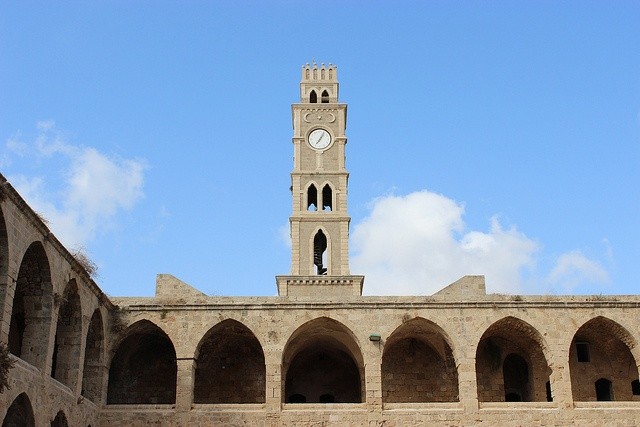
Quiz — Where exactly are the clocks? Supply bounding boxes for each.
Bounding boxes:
[307,127,333,150]
